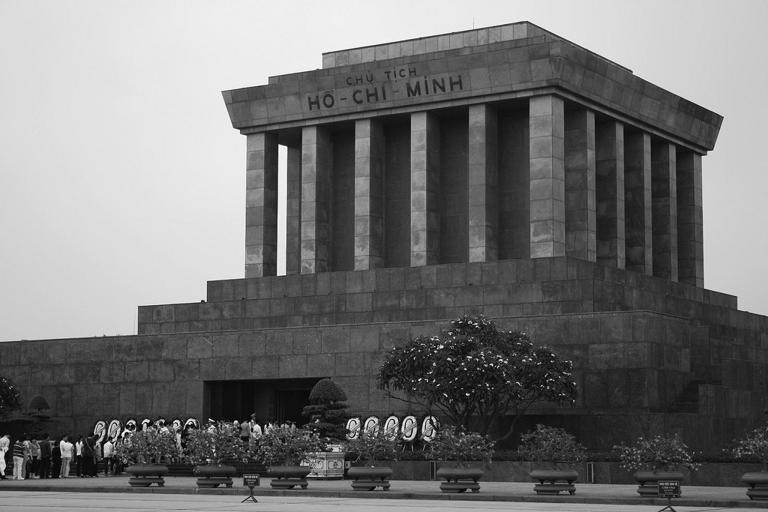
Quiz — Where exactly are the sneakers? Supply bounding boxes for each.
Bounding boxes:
[0,475,40,480]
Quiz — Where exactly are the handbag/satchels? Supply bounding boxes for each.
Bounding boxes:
[91,450,96,455]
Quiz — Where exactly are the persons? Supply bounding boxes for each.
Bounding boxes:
[1,408,299,489]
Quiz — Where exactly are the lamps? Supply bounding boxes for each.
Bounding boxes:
[656,480,682,512]
[238,473,260,503]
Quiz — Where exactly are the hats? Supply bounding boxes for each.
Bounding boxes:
[250,413,257,417]
[208,418,215,423]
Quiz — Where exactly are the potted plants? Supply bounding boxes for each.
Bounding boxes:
[120,428,178,488]
[189,425,241,490]
[611,431,703,498]
[299,378,350,480]
[265,442,311,491]
[421,424,497,493]
[513,421,587,496]
[721,424,767,500]
[348,429,399,495]
